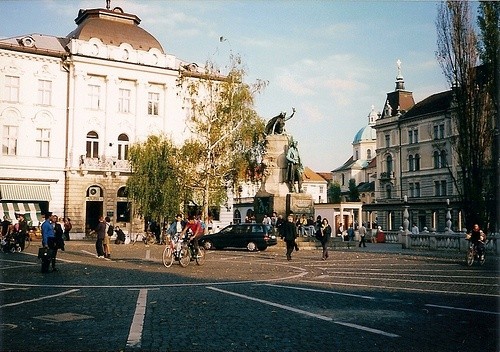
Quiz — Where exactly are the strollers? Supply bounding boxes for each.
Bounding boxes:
[0,230,22,254]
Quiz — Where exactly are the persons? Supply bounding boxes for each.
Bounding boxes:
[467,224,484,261]
[270,107,304,193]
[0,211,419,272]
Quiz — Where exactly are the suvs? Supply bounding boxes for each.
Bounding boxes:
[197,223,278,252]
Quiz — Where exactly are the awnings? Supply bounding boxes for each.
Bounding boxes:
[0,184,52,202]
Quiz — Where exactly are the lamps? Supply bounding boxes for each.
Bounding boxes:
[81,155,85,164]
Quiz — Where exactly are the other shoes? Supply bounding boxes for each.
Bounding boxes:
[325,256,329,259]
[322,258,325,260]
[286,254,291,260]
[104,258,111,261]
[96,255,104,258]
[481,255,484,260]
[53,268,59,271]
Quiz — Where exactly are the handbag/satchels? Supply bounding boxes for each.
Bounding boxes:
[38,248,51,259]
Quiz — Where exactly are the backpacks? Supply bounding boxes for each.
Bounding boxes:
[106,223,113,236]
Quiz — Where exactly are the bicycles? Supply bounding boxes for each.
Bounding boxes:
[162,232,207,268]
[465,238,486,266]
